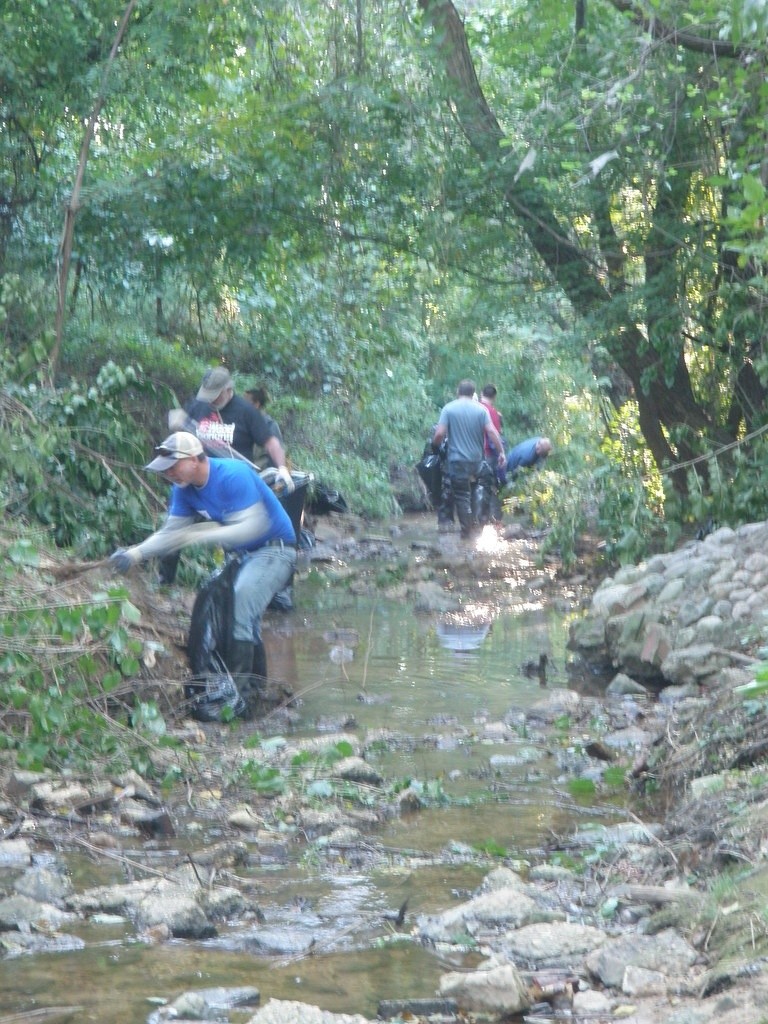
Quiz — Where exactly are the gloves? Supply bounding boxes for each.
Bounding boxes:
[109,546,140,574]
[275,465,295,494]
[168,409,188,432]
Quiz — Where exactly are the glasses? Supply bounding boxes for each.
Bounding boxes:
[154,446,192,457]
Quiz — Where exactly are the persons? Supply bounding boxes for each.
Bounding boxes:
[107,367,296,722]
[435,374,554,540]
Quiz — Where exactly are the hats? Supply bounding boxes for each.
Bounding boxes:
[142,431,203,471]
[195,366,230,403]
